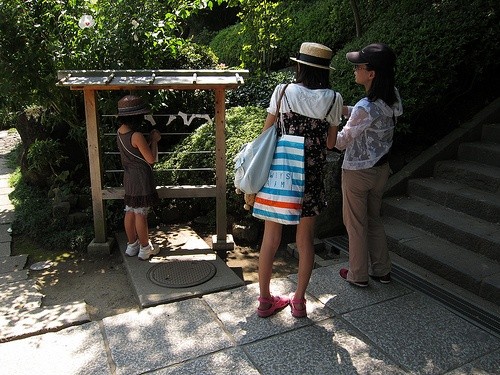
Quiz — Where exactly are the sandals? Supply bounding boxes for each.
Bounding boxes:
[289,292,307,317]
[258,295,290,318]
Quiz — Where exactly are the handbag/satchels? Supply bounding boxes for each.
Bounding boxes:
[233,124,277,194]
[252,134,305,225]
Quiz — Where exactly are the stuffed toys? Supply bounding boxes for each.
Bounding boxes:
[235,189,256,210]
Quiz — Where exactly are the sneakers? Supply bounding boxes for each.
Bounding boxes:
[125,238,140,257]
[138,241,160,261]
[340,269,368,286]
[369,272,393,283]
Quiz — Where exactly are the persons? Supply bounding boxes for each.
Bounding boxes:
[335,43,402,287]
[116,95,160,259]
[257,42,343,319]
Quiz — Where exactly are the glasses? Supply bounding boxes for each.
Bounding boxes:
[355,65,368,71]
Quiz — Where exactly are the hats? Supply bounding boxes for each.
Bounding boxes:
[290,41,337,71]
[346,43,396,72]
[114,95,155,116]
